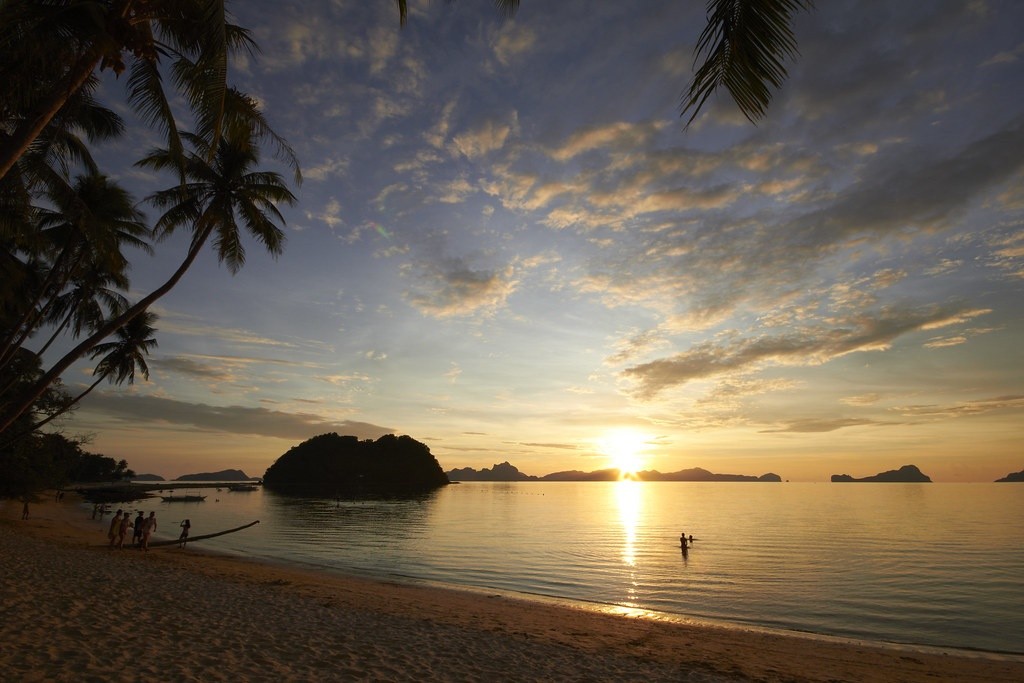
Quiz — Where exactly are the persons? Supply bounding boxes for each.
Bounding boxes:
[689,535,693,542]
[681,532,689,551]
[140,512,158,552]
[178,519,191,551]
[99,501,107,521]
[108,509,123,550]
[93,501,102,520]
[22,497,33,520]
[116,512,134,551]
[54,490,66,505]
[132,511,146,548]
